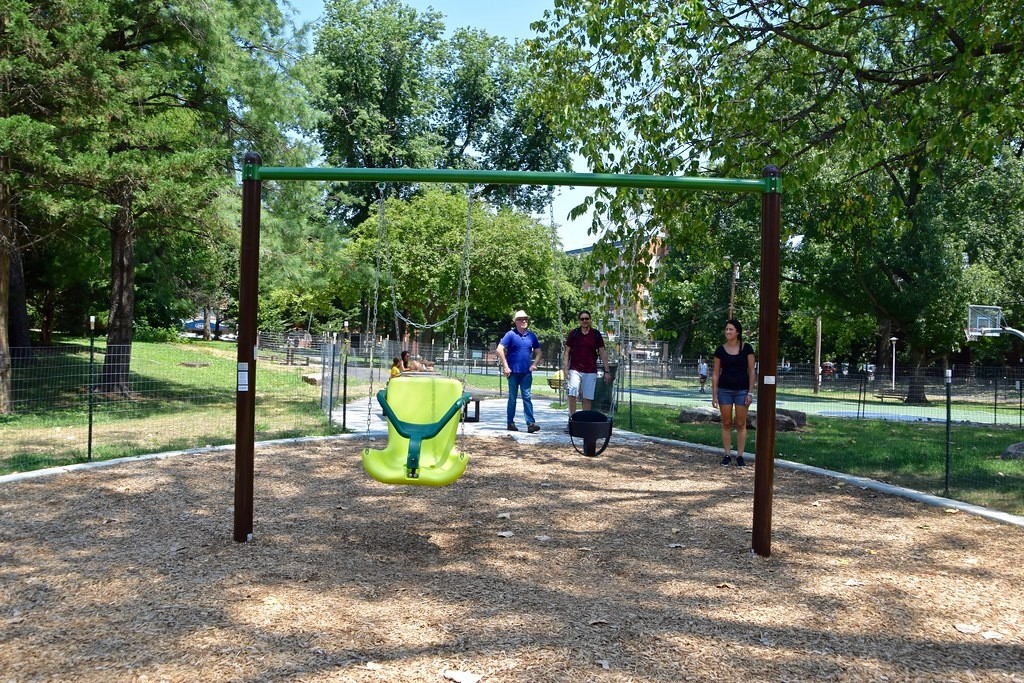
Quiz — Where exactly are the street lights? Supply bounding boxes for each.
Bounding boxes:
[889,336,899,392]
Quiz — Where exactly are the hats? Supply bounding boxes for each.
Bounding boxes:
[513,310,530,322]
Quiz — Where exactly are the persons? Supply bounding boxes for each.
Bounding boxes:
[698,358,709,394]
[868,366,874,373]
[390,352,435,378]
[288,327,312,348]
[496,310,542,433]
[713,319,755,467]
[564,311,611,433]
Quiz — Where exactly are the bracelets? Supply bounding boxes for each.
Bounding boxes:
[605,371,610,374]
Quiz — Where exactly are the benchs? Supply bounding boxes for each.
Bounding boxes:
[546,378,565,393]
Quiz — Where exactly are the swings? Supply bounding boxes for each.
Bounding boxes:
[360,189,474,486]
[545,190,646,457]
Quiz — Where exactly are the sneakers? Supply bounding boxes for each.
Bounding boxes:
[736,456,747,468]
[720,455,731,466]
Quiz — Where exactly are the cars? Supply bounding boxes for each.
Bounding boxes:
[822,360,875,379]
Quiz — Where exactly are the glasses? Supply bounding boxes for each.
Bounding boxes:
[517,319,528,323]
[579,317,590,321]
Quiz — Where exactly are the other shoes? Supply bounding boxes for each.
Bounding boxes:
[528,423,540,433]
[507,423,518,431]
[700,390,705,394]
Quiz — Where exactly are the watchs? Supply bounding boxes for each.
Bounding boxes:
[748,393,754,397]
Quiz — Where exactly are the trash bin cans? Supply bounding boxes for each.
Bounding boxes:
[582,361,618,412]
